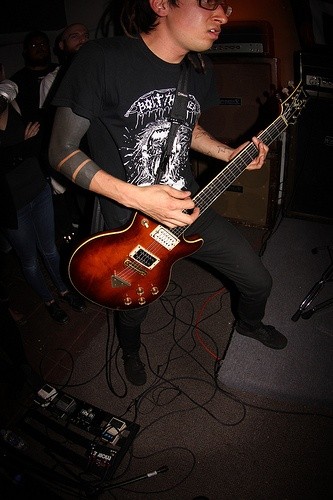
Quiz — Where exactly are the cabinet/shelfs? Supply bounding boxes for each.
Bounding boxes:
[190,58,279,228]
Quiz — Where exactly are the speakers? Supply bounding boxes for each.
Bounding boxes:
[189,148,276,231]
[274,90,333,225]
[198,57,277,149]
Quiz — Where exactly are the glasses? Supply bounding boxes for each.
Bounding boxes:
[198,0,232,17]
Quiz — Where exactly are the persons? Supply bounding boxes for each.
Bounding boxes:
[0,62,87,325]
[36,10,118,311]
[21,32,60,83]
[45,0,290,385]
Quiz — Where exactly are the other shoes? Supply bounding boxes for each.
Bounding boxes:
[122,348,146,386]
[45,299,67,322]
[235,320,288,350]
[60,289,86,313]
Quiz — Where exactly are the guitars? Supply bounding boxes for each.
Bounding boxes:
[67,76,308,312]
